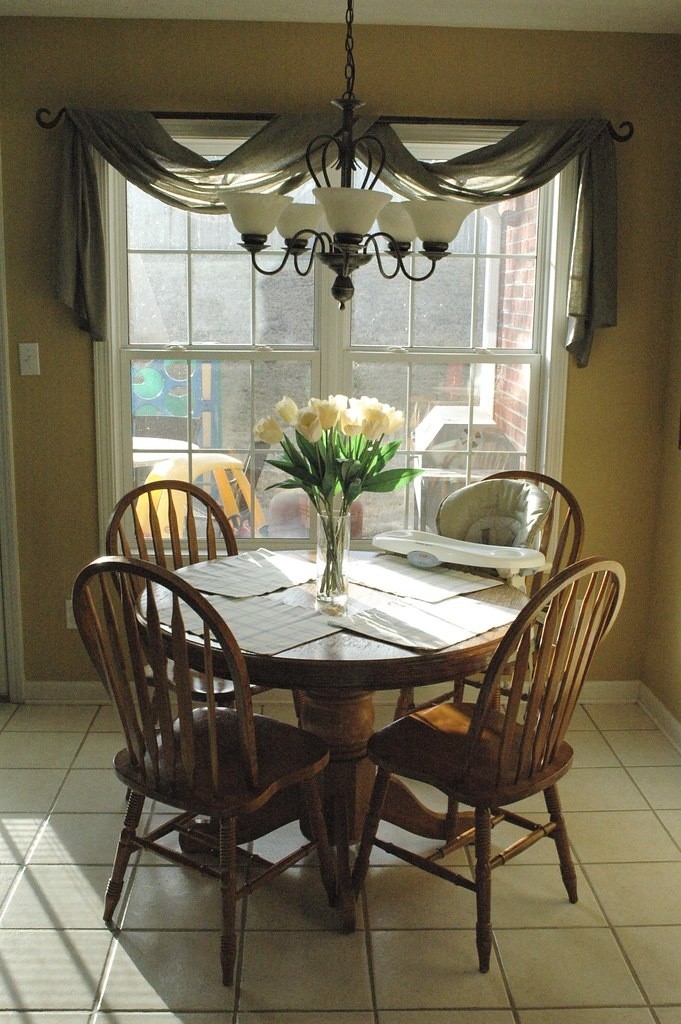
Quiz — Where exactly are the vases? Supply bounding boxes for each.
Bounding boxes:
[315,509,351,614]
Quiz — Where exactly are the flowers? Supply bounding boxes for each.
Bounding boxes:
[251,390,425,597]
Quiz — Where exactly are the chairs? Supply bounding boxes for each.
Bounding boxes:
[69,554,342,987]
[104,478,272,716]
[391,470,585,724]
[349,555,627,974]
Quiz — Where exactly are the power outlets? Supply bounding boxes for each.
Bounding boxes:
[18,342,40,376]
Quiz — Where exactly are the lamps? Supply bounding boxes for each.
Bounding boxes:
[218,0,477,313]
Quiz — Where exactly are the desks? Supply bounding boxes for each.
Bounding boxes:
[135,547,538,848]
